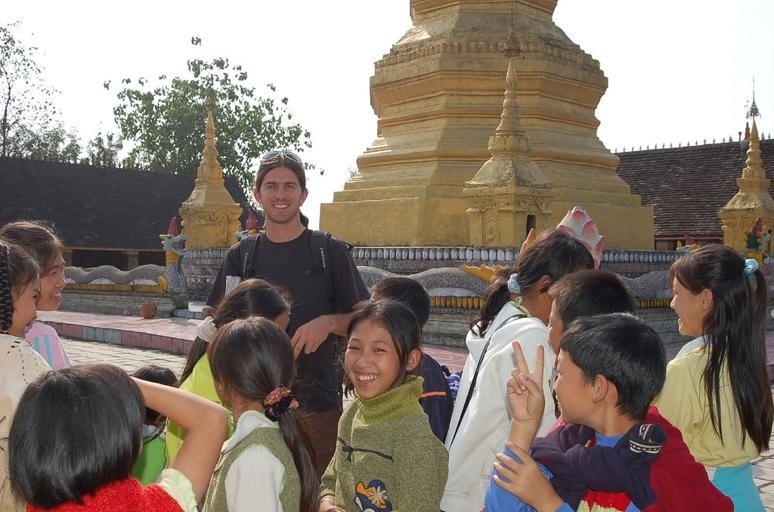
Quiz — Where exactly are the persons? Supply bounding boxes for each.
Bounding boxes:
[205,148,373,474]
[0,219,774,512]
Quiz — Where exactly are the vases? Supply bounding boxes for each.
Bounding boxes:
[140,304,157,319]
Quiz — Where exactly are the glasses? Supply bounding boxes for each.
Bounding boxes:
[260,151,302,167]
